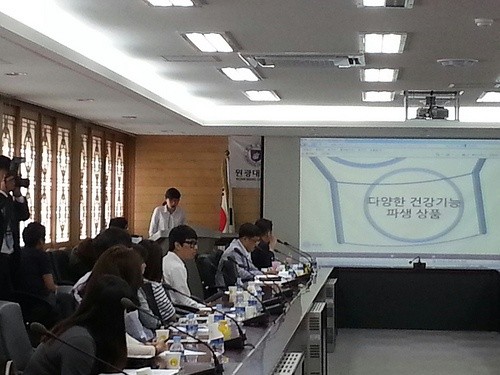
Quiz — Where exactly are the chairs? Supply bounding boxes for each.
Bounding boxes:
[0,245,224,375]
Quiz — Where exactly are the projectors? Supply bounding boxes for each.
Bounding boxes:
[416,108,449,119]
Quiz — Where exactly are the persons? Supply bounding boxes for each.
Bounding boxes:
[214,222,263,292]
[24,275,134,375]
[5,221,55,345]
[148,187,185,238]
[72,216,177,361]
[252,218,276,269]
[161,223,212,315]
[0,154,29,265]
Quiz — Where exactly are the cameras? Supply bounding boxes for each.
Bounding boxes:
[4,157,30,188]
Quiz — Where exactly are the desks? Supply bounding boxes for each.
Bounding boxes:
[329,267,500,331]
[148,267,338,375]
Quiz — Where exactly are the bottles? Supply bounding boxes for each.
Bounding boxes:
[186,313,199,339]
[235,292,245,317]
[214,303,225,322]
[288,269,295,280]
[169,336,185,369]
[218,319,229,341]
[256,283,263,310]
[246,282,258,318]
[235,278,244,291]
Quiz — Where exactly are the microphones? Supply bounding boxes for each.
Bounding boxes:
[120,239,313,375]
[29,322,128,375]
[409,256,426,269]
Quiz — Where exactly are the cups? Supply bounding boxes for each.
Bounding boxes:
[155,286,255,370]
[272,262,280,271]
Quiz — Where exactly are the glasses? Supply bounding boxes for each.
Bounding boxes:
[247,237,261,245]
[166,197,180,202]
[184,241,199,248]
[125,225,129,231]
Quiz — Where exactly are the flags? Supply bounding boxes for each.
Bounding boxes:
[227,135,261,187]
[218,150,236,234]
